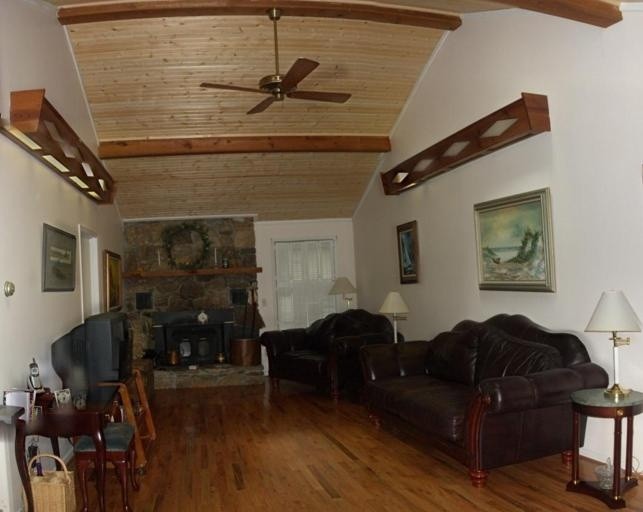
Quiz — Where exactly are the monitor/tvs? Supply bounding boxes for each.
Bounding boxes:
[52,312,132,389]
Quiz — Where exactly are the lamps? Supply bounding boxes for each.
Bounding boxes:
[378,291,412,345]
[583,288,641,400]
[327,277,360,308]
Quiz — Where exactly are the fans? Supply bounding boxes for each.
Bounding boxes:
[198,4,354,116]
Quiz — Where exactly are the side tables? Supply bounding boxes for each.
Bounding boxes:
[565,387,643,511]
[10,384,123,512]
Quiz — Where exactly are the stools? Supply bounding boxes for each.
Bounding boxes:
[72,420,141,512]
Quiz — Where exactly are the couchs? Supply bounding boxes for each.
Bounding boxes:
[358,312,610,488]
[257,307,406,401]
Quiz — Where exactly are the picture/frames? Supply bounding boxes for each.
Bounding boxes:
[473,187,556,293]
[397,220,419,284]
[41,222,76,292]
[103,249,122,312]
